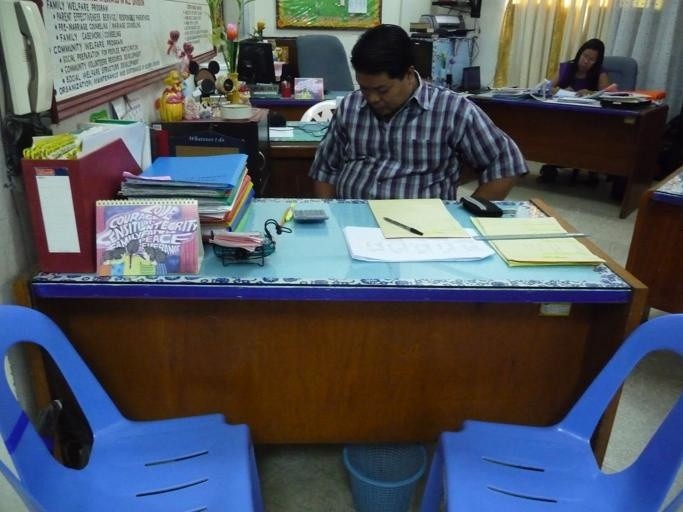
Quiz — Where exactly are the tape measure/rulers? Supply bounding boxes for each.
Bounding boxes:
[473,232,590,241]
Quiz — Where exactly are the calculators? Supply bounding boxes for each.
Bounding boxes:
[293,209,328,223]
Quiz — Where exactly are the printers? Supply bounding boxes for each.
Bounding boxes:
[420,14,476,38]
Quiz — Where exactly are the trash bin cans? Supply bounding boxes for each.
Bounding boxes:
[342,443,428,512]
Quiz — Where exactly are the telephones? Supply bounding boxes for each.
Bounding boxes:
[0,0,54,116]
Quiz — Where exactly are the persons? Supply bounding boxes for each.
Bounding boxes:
[539,39,610,177]
[308,24,529,200]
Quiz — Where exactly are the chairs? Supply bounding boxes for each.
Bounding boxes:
[0,305,266,512]
[296,34,356,91]
[420,314,683,512]
[601,56,638,91]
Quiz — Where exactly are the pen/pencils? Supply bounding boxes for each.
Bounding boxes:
[383,217,423,235]
[284,202,295,222]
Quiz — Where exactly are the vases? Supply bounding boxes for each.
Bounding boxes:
[227,72,241,104]
[440,67,447,80]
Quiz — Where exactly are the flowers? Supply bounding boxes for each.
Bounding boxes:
[438,52,455,68]
[203,11,240,73]
[247,20,266,42]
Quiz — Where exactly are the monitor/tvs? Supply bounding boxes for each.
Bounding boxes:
[230,40,277,86]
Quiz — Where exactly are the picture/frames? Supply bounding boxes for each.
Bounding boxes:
[276,0,382,30]
